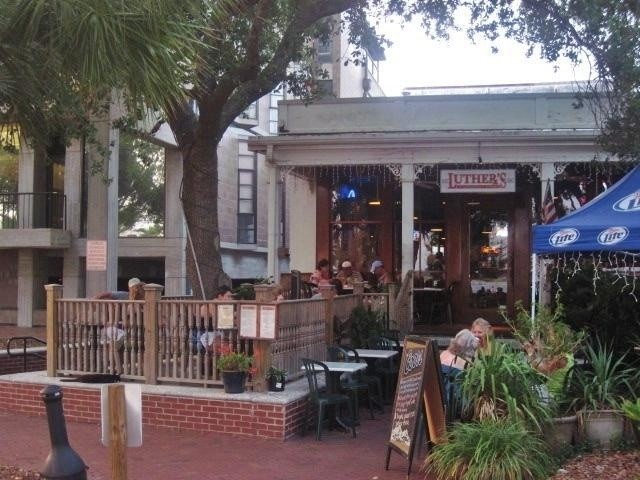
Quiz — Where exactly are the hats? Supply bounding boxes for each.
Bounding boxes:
[369,260,384,272]
[342,260,352,268]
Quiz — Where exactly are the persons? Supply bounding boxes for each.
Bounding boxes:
[438,326,480,420]
[184,284,235,376]
[92,324,129,376]
[470,317,493,351]
[519,322,576,411]
[273,255,396,353]
[92,276,147,353]
[413,245,507,310]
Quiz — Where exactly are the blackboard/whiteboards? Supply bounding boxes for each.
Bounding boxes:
[388,335,434,458]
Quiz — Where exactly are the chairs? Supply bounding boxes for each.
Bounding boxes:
[303,282,318,298]
[429,280,460,328]
[300,329,405,441]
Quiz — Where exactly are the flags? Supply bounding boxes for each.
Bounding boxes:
[541,178,560,225]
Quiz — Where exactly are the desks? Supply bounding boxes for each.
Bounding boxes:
[413,287,445,324]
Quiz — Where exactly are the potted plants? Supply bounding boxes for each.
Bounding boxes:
[264,365,289,391]
[253,273,279,301]
[540,328,640,452]
[217,351,254,393]
